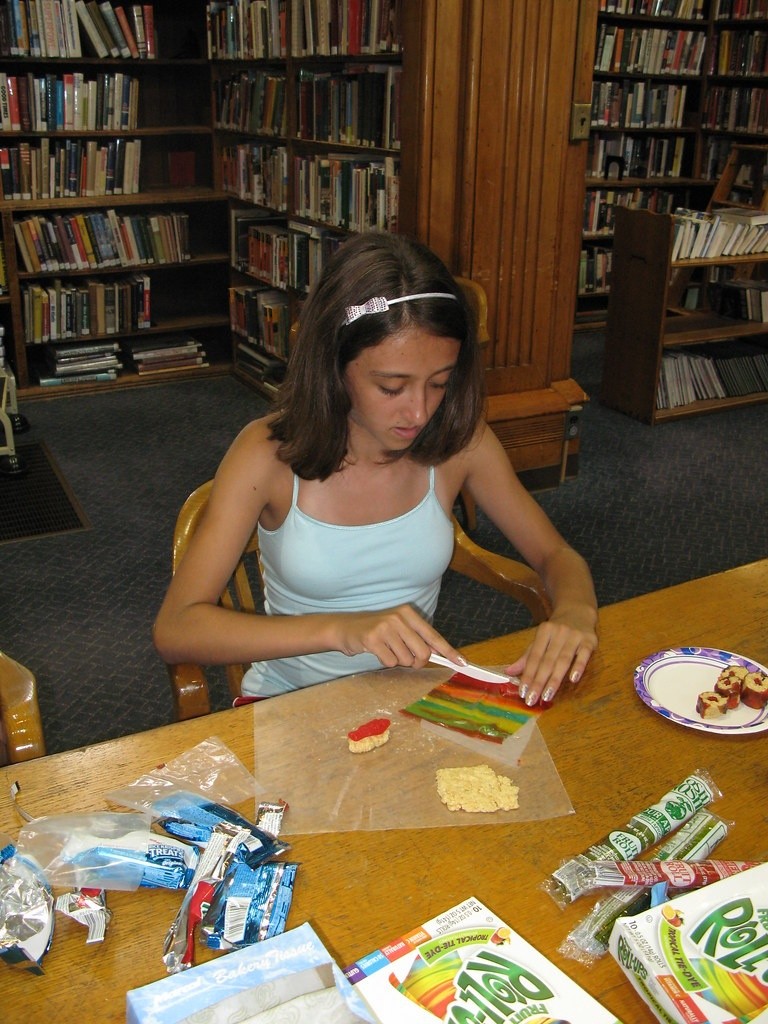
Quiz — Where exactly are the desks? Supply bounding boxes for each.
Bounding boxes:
[0,561,768,1024]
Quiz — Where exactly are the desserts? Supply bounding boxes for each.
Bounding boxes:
[348,719,390,753]
[697,665,768,720]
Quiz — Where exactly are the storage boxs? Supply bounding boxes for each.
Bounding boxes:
[341,896,623,1024]
[126,921,377,1024]
[609,861,768,1024]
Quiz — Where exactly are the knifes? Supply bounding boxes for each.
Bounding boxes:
[428,652,510,684]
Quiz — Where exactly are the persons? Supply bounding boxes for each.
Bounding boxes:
[154,234,597,707]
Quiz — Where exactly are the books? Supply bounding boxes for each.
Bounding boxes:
[0,0,208,387]
[577,1,768,409]
[206,0,401,393]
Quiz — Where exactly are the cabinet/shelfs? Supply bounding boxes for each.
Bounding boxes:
[571,0,712,335]
[0,0,233,406]
[206,0,436,404]
[699,19,768,193]
[596,203,768,427]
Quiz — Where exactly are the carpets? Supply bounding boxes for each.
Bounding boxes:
[0,439,98,546]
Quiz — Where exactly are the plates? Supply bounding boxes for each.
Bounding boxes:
[633,647,768,735]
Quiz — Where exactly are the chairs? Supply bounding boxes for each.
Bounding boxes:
[167,479,553,722]
